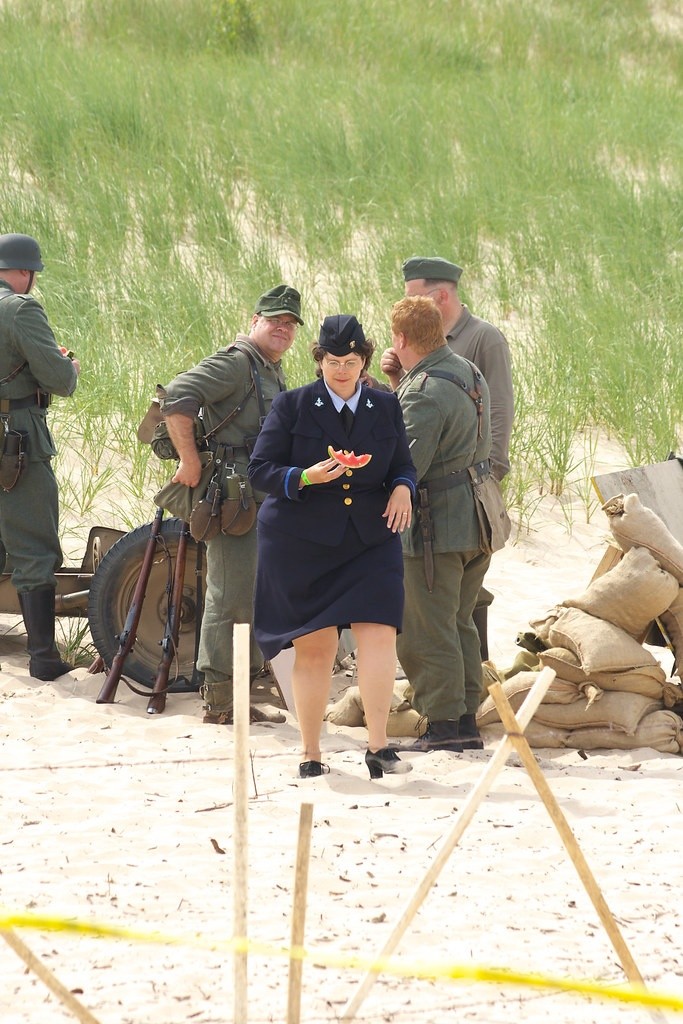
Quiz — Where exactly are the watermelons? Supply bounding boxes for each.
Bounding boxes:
[328,445,372,469]
[58,346,75,358]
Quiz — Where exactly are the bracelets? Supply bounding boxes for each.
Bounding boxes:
[301,470,313,486]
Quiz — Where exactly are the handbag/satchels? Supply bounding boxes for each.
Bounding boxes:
[150,415,206,459]
[153,451,215,522]
[468,467,511,555]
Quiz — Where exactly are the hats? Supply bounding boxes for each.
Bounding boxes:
[255,284,304,326]
[319,315,365,357]
[402,256,462,281]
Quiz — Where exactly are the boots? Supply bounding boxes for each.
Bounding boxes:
[18,586,76,681]
[200,679,286,725]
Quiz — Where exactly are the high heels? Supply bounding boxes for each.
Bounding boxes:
[364,746,412,779]
[299,760,331,778]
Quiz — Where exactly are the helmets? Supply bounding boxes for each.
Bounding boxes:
[0,233,43,271]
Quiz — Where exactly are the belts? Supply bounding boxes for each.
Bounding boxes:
[9,392,52,410]
[415,458,489,494]
[209,444,248,458]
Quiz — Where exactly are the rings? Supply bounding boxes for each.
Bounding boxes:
[403,512,408,515]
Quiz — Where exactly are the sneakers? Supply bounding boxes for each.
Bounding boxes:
[389,720,463,752]
[459,714,484,749]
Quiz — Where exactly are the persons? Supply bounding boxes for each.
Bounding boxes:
[361,257,513,752]
[161,286,304,726]
[0,234,80,682]
[248,315,416,779]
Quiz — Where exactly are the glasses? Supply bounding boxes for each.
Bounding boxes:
[264,318,298,330]
[323,360,361,370]
